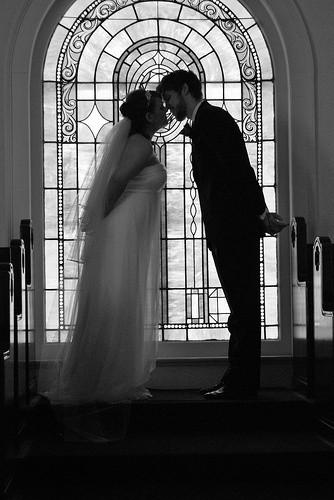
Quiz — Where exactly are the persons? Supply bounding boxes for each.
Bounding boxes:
[36,90,169,406]
[156,69,290,403]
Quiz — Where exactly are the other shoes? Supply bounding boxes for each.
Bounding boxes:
[199,380,256,399]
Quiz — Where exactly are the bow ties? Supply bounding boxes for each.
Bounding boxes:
[179,122,198,138]
